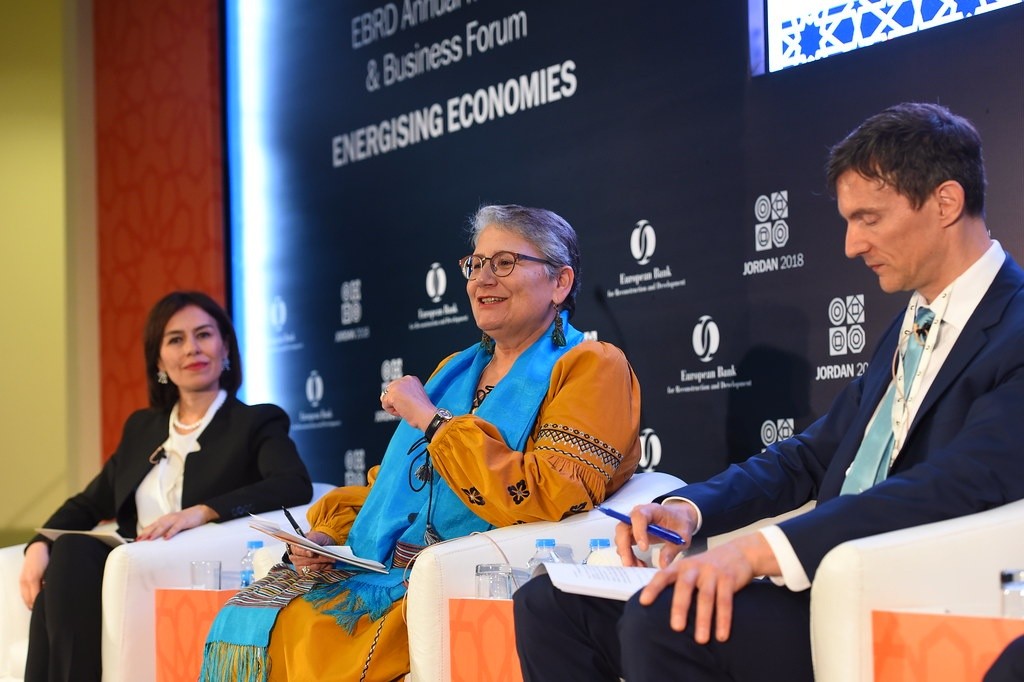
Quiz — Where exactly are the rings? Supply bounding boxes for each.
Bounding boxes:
[301,565,310,574]
[285,543,292,555]
[381,389,388,397]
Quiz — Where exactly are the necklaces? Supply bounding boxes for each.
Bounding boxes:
[174,415,203,430]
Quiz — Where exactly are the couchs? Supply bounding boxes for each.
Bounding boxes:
[0,478,336,677]
[101,470,685,682]
[587,496,1024,682]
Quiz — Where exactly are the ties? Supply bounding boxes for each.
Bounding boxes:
[840,306,936,498]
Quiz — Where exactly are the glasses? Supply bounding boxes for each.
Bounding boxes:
[458,251,557,280]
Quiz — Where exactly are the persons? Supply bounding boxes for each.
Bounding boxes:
[201,203,639,682]
[20,291,313,682]
[512,103,1024,682]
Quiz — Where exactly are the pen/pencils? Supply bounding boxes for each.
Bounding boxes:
[593,503,688,546]
[281,505,307,539]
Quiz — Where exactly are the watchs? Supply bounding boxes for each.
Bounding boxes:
[424,408,453,441]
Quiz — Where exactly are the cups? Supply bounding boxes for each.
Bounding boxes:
[190,561,222,590]
[1002,571,1024,619]
[476,565,512,601]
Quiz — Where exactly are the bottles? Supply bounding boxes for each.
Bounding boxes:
[241,541,264,589]
[527,538,565,576]
[583,539,612,568]
